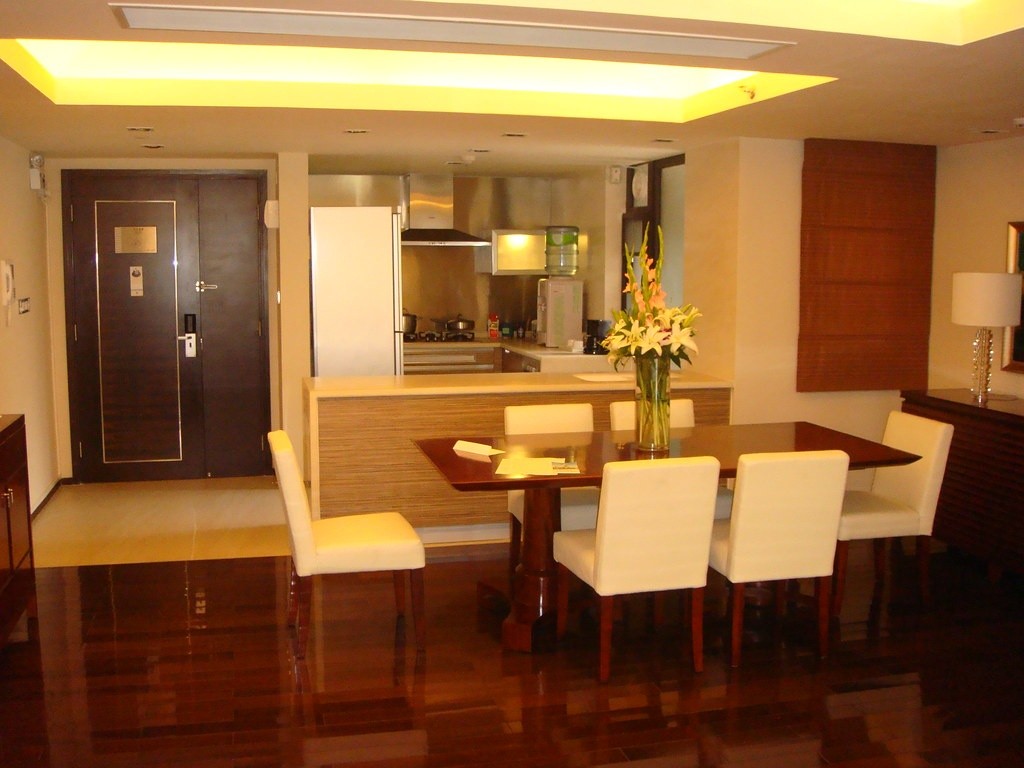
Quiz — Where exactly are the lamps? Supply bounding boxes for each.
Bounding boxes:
[952,272,1023,408]
[29,151,50,205]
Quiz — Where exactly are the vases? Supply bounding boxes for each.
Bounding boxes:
[636,355,672,453]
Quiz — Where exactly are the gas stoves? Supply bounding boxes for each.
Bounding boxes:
[403,332,478,346]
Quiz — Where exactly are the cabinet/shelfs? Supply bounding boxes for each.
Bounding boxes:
[403,348,542,376]
[901,388,1024,561]
[0,412,35,592]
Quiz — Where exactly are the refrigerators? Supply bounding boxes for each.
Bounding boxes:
[310,204,404,377]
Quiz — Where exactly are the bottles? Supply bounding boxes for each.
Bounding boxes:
[487,312,499,340]
[545,225,579,276]
[516,323,526,338]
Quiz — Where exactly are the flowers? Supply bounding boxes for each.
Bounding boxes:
[602,219,703,371]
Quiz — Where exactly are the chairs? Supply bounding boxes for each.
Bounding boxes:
[608,400,735,521]
[830,412,955,616]
[268,430,426,660]
[686,450,852,666]
[503,403,602,582]
[552,455,720,681]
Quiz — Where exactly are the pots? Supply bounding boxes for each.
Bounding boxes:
[401,307,423,334]
[430,313,475,332]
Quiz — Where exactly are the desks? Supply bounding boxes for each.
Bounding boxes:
[410,422,922,655]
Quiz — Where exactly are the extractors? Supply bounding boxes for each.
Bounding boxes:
[401,173,492,247]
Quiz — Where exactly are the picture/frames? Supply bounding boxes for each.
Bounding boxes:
[1002,220,1024,373]
